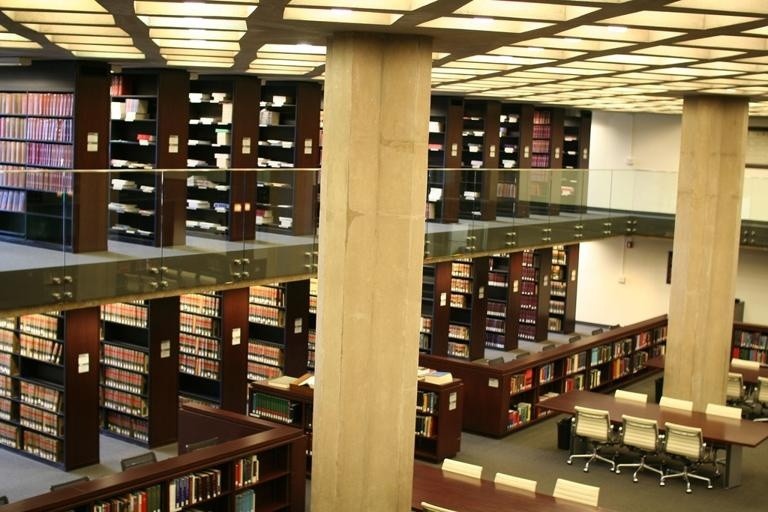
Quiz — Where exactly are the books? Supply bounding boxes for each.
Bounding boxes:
[179,292,221,380]
[186,92,233,232]
[1,93,74,212]
[461,116,485,216]
[560,135,580,196]
[249,282,284,327]
[507,352,584,430]
[416,367,453,436]
[0,307,63,463]
[578,321,768,392]
[486,246,566,349]
[309,278,318,313]
[256,96,294,229]
[419,258,472,358]
[247,340,315,424]
[530,110,552,196]
[425,121,444,220]
[99,300,150,442]
[308,330,317,368]
[109,99,156,237]
[497,114,519,197]
[88,451,265,511]
